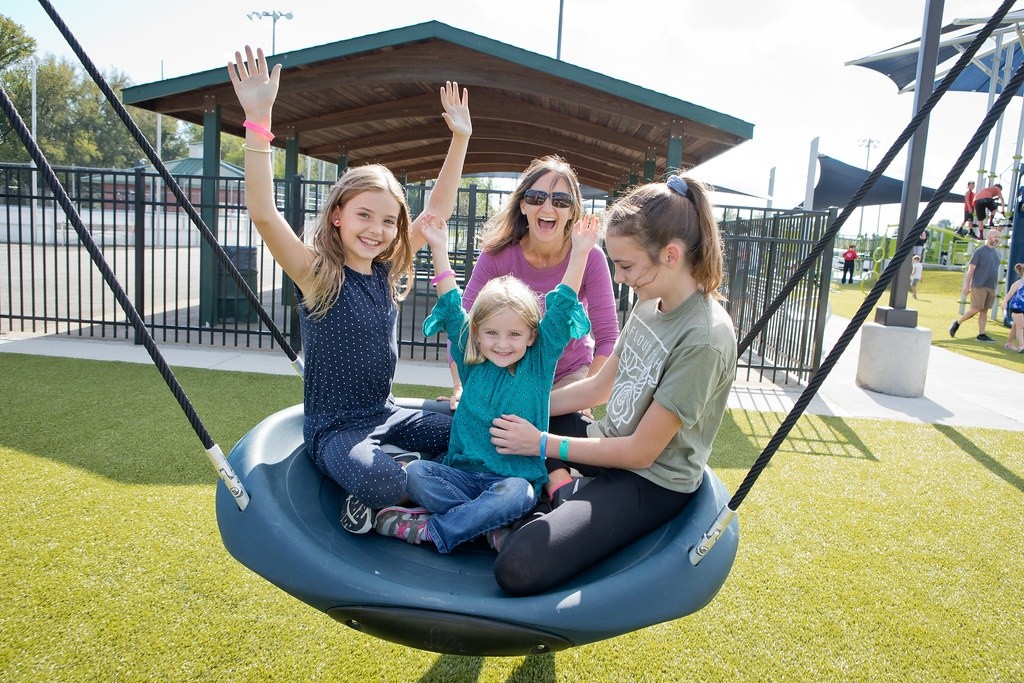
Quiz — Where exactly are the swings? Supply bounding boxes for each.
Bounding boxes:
[0,0,1024,659]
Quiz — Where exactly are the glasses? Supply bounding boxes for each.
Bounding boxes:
[522,189,572,208]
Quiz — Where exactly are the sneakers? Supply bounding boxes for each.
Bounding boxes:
[485,528,512,554]
[372,506,438,544]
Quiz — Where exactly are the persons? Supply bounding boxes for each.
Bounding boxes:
[972,184,1004,240]
[436,153,620,420]
[479,174,739,596]
[228,45,472,534]
[912,232,926,261]
[841,244,858,283]
[949,229,1001,342]
[910,255,922,299]
[373,213,601,552]
[955,181,976,235]
[1001,263,1024,353]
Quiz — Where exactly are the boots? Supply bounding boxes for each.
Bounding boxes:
[977,230,984,239]
[989,215,994,226]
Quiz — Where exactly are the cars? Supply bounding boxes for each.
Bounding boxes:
[831,247,847,271]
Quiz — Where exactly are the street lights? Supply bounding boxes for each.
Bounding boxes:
[856,138,881,238]
[246,11,296,178]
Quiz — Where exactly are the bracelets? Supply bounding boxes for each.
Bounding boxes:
[242,120,275,141]
[559,438,569,461]
[539,431,547,460]
[432,270,457,287]
[241,144,272,153]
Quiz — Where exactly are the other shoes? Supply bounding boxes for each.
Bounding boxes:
[966,232,977,238]
[955,230,964,237]
[1005,344,1024,353]
[537,475,595,511]
[975,333,995,344]
[950,320,960,337]
[339,492,377,535]
